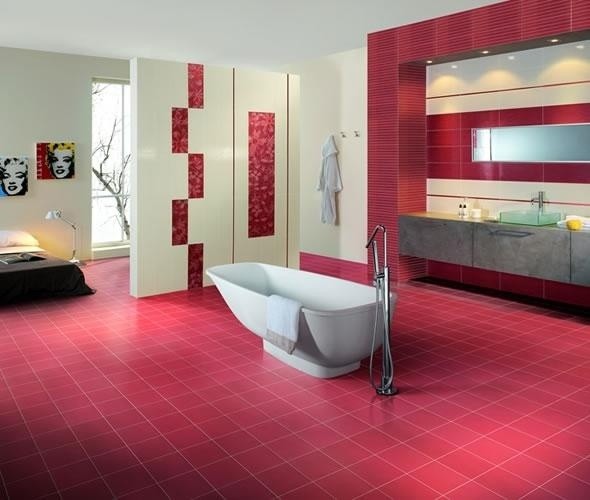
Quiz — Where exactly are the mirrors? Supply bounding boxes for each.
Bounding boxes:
[469,124,590,164]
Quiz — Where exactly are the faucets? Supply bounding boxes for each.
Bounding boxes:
[531,192,543,208]
[366,224,388,266]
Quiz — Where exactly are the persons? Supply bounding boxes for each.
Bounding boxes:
[46,143,74,179]
[0,156,27,196]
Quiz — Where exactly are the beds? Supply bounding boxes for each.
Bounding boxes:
[0,230,87,306]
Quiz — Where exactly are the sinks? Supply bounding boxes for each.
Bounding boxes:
[500,211,560,226]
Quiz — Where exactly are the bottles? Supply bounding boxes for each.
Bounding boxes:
[458,201,468,219]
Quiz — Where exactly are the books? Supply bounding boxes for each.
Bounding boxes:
[0,252,47,265]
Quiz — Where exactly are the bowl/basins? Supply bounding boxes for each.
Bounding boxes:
[566,220,581,231]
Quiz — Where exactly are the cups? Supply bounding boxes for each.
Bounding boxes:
[471,209,481,219]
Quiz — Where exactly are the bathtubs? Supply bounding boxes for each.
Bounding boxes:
[205,262,397,379]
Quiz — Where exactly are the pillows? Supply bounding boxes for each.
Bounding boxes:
[260,293,301,354]
[0,230,39,248]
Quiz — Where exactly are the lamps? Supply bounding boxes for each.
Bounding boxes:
[44,210,81,263]
